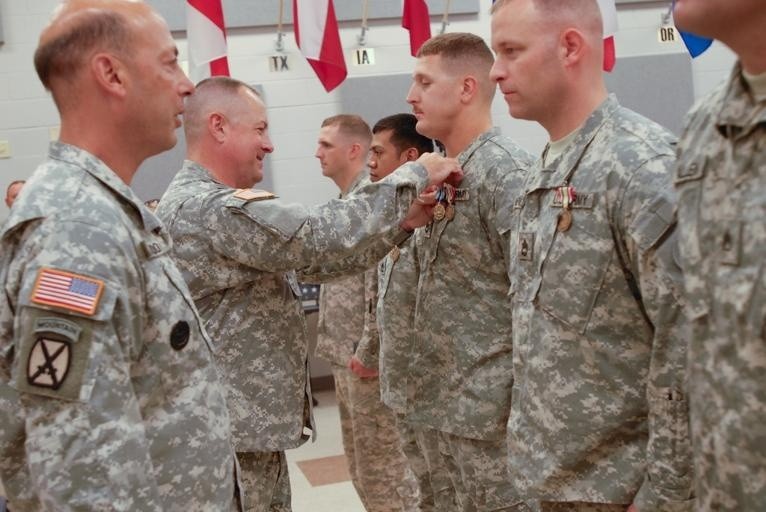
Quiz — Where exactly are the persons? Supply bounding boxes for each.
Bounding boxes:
[368,111,459,511]
[4,176,28,208]
[487,0,698,511]
[0,0,246,512]
[152,75,465,511]
[311,113,423,510]
[670,1,766,511]
[405,30,539,512]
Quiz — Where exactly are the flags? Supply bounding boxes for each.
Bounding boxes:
[596,0,619,73]
[292,0,349,94]
[401,0,432,58]
[672,0,716,60]
[183,1,231,90]
